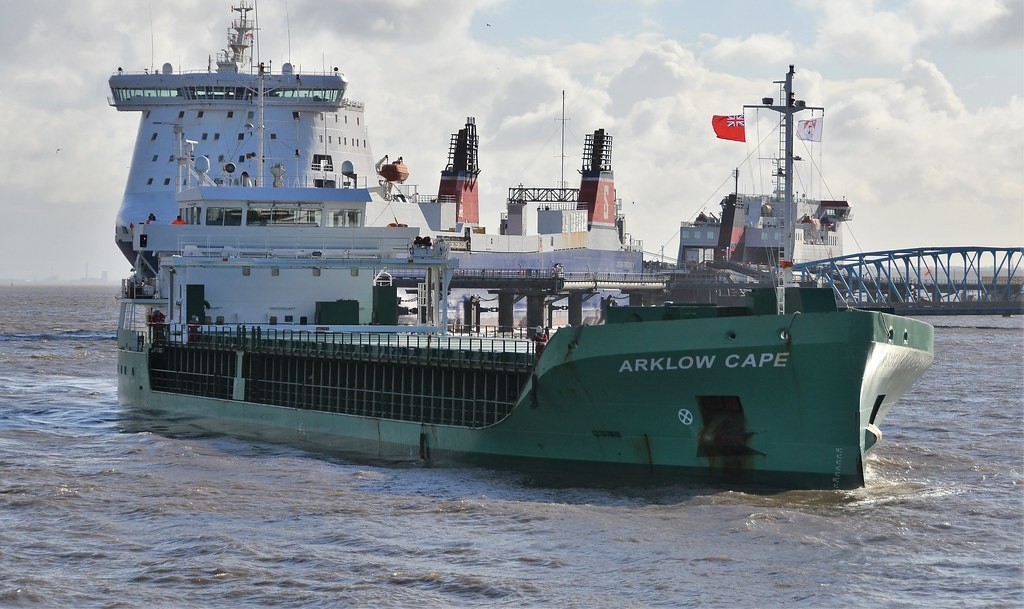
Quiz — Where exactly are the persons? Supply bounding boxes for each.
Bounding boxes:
[188,315,200,342]
[146,213,156,224]
[150,310,166,339]
[172,215,185,224]
[530,326,548,357]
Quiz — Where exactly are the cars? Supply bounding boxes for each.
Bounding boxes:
[106,2,937,489]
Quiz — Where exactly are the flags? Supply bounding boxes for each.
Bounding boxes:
[795,117,824,142]
[712,115,746,143]
